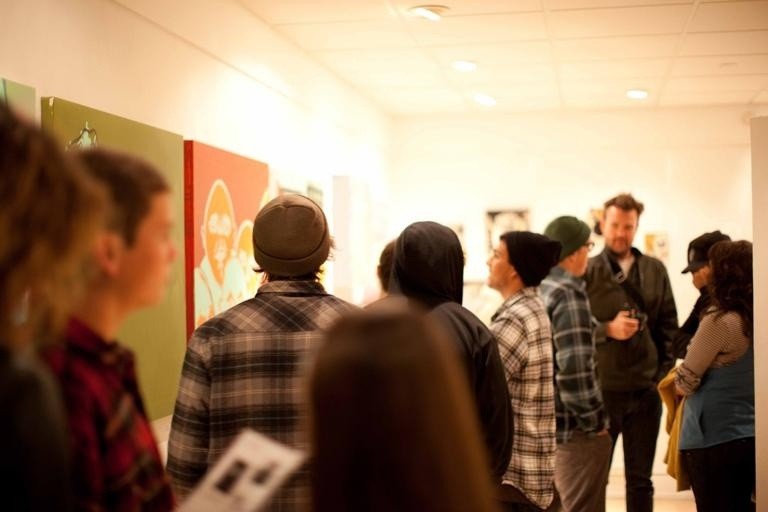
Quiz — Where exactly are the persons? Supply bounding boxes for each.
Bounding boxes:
[672,239,753,512]
[359,242,413,318]
[669,231,731,367]
[308,313,535,509]
[582,195,679,512]
[1,99,113,511]
[487,232,564,508]
[166,193,363,511]
[38,149,179,509]
[363,222,514,479]
[538,216,613,512]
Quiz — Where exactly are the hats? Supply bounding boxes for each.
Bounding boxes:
[250,193,331,277]
[503,229,564,288]
[681,229,733,275]
[542,216,592,263]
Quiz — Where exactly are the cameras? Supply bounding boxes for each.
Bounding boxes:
[625,306,648,331]
